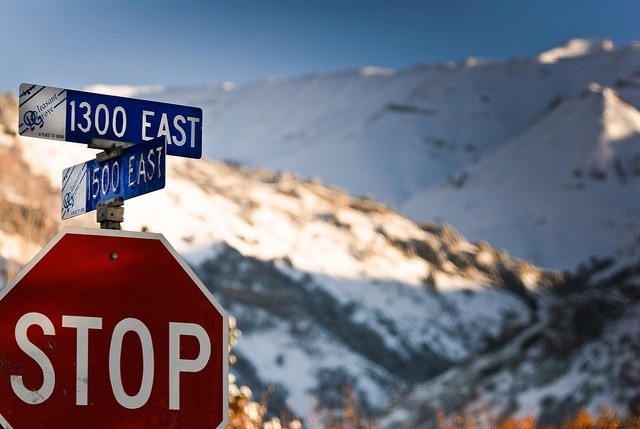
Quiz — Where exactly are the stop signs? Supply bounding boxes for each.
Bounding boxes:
[0,227,228,429]
